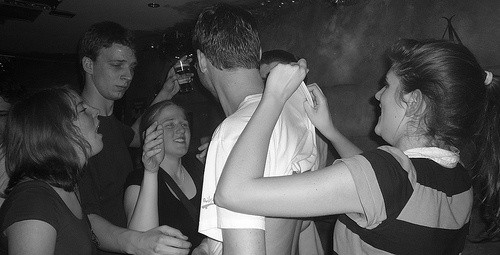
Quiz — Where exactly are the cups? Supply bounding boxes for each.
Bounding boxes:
[174,59,193,92]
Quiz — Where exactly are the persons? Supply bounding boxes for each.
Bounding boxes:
[195,5,328,255]
[123,101,210,255]
[215,40,500,255]
[0,25,193,255]
